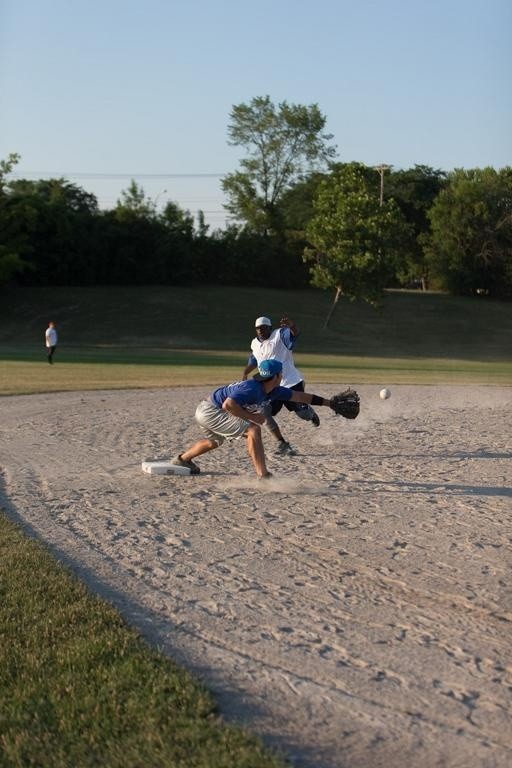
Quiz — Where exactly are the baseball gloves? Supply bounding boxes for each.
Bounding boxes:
[330,387,360,419]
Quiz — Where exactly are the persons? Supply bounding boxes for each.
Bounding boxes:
[45,322,58,364]
[172,359,361,481]
[241,315,320,455]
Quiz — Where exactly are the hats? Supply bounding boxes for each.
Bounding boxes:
[255,317,271,328]
[253,360,282,382]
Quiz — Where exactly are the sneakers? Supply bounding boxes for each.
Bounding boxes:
[275,441,290,453]
[173,455,200,473]
[311,414,320,426]
[258,471,273,481]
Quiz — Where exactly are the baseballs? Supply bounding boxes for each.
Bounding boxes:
[380,389,391,400]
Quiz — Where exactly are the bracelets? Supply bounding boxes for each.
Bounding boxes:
[311,394,324,406]
